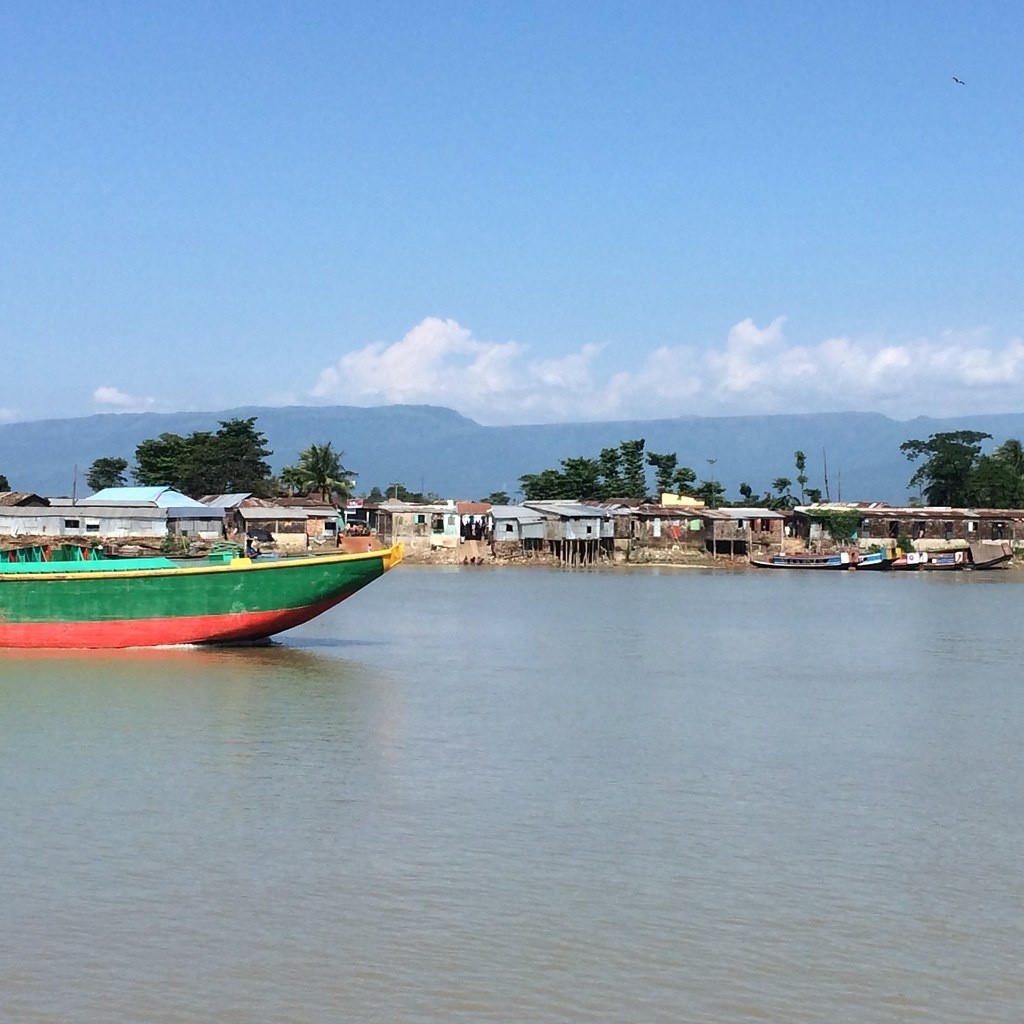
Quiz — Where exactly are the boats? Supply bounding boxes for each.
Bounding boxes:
[748,551,867,569]
[1,516,405,648]
[856,541,1015,570]
